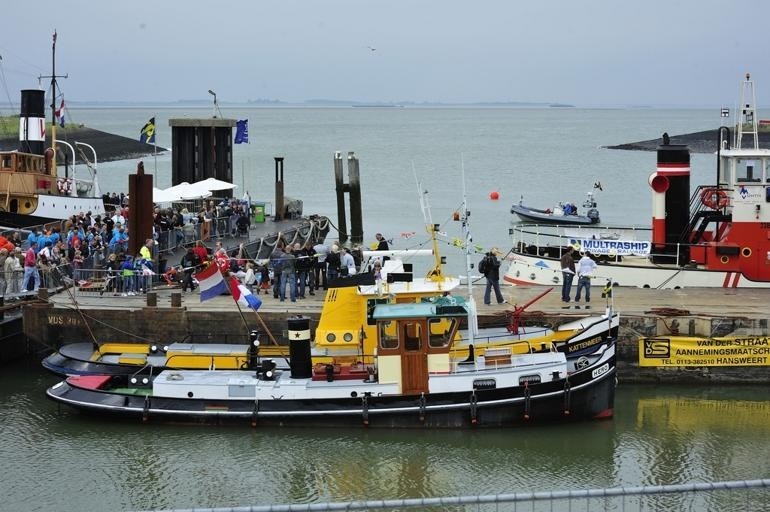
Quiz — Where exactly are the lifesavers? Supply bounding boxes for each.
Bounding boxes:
[701,189,727,210]
[57,178,72,193]
[215,256,230,272]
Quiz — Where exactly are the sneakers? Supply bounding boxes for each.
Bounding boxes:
[488,299,507,305]
[273,287,328,302]
[34,288,39,292]
[20,289,28,293]
[113,287,143,297]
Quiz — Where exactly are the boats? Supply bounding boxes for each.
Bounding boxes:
[501,72,770,291]
[0,30,126,236]
[40,154,621,429]
[510,180,602,225]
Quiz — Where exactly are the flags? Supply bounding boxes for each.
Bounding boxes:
[138,116,156,143]
[358,325,369,348]
[601,281,613,298]
[228,275,263,313]
[232,118,249,144]
[53,100,65,128]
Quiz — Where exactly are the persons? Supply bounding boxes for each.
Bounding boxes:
[0,188,390,303]
[573,250,599,303]
[560,246,576,302]
[482,247,508,305]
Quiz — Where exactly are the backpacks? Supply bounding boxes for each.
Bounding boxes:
[479,253,494,274]
[296,252,312,271]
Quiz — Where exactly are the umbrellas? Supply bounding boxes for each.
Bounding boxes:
[126,187,183,202]
[163,181,213,200]
[191,177,238,190]
